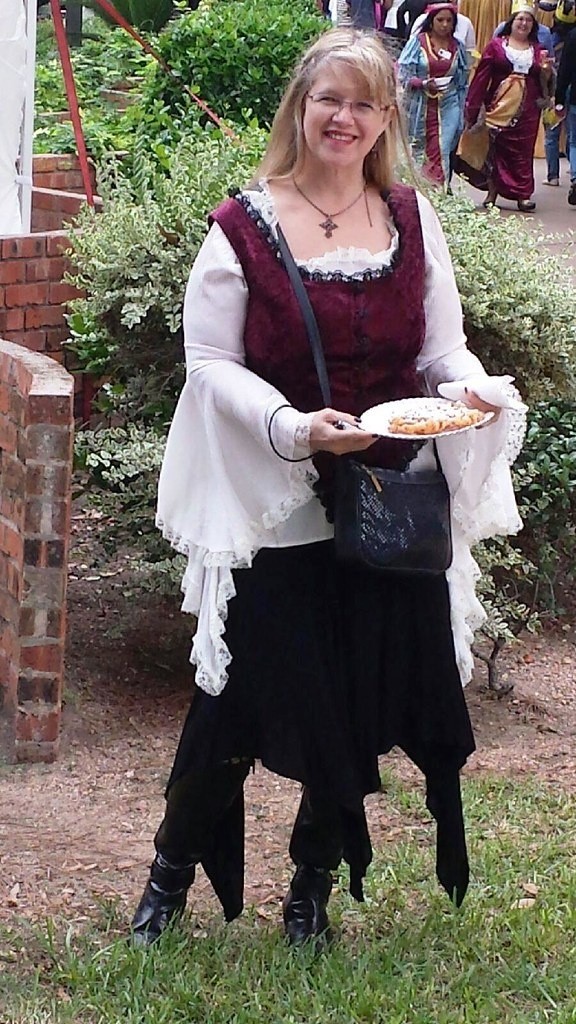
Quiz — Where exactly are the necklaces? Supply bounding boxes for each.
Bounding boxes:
[292,171,368,240]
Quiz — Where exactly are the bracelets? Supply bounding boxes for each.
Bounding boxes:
[268,405,322,463]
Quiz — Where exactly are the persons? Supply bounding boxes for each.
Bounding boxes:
[125,26,529,964]
[451,0,576,212]
[329,0,481,196]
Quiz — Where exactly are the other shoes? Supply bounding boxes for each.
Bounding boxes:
[543,177,559,186]
[483,191,496,208]
[567,182,576,205]
[518,199,535,210]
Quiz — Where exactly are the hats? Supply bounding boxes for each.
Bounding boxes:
[511,0,538,18]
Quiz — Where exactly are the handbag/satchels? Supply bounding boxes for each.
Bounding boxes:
[334,459,452,573]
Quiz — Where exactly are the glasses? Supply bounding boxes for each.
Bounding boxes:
[306,91,388,120]
[512,16,533,25]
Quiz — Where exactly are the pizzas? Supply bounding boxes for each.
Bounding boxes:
[389,401,484,435]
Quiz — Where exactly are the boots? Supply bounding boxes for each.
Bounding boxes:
[281,861,333,956]
[128,859,197,953]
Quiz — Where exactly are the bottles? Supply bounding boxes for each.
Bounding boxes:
[555,103,564,118]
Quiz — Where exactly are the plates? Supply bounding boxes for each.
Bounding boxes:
[358,397,495,439]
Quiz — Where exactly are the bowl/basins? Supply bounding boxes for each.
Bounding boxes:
[434,76,453,89]
